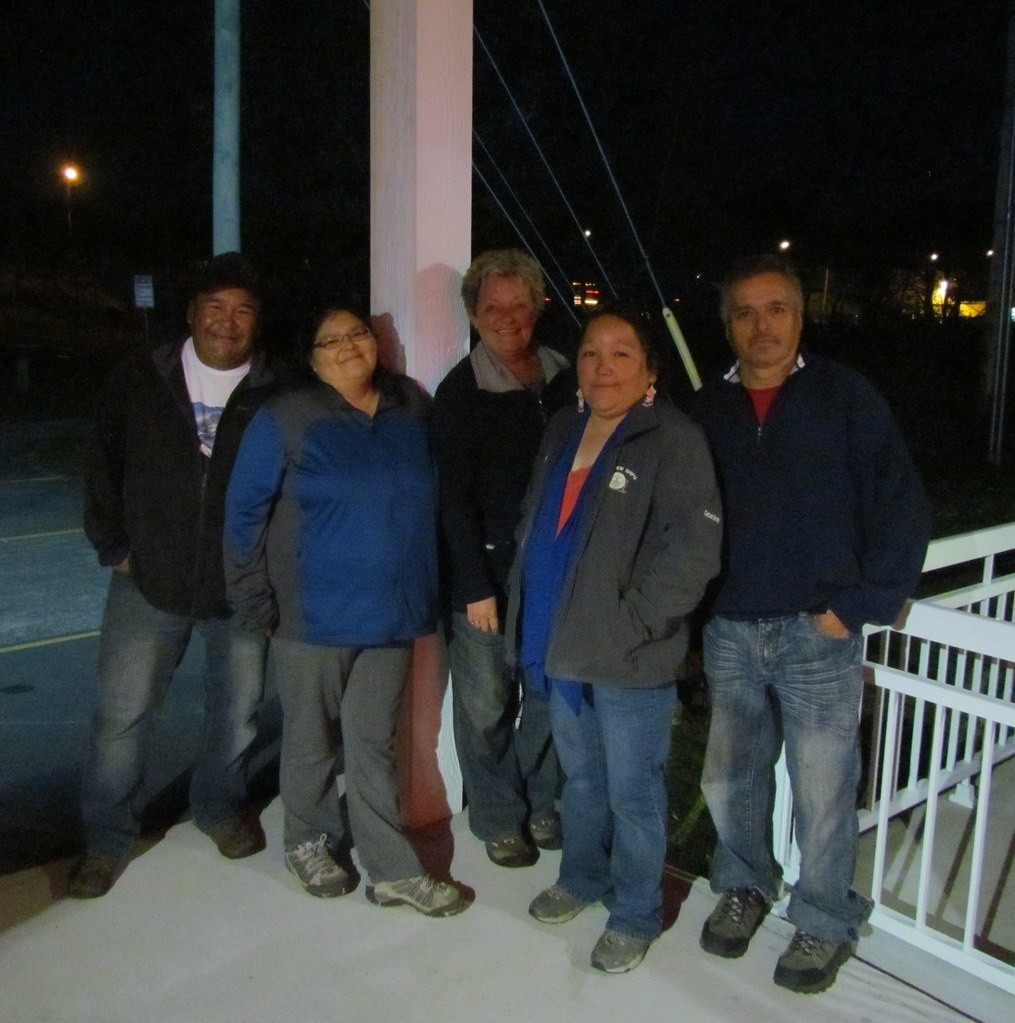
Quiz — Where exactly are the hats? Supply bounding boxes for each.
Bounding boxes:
[186,252,267,302]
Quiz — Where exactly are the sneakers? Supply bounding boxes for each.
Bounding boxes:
[285,833,353,898]
[774,928,858,994]
[591,928,660,974]
[485,832,537,867]
[65,846,129,898]
[365,872,467,917]
[529,885,613,924]
[194,808,267,859]
[699,886,773,959]
[528,813,563,850]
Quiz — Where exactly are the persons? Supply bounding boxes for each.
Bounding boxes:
[75,252,295,897]
[504,306,721,975]
[223,304,463,917]
[433,249,577,866]
[682,250,930,992]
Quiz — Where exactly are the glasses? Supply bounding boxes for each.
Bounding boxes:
[311,323,371,352]
